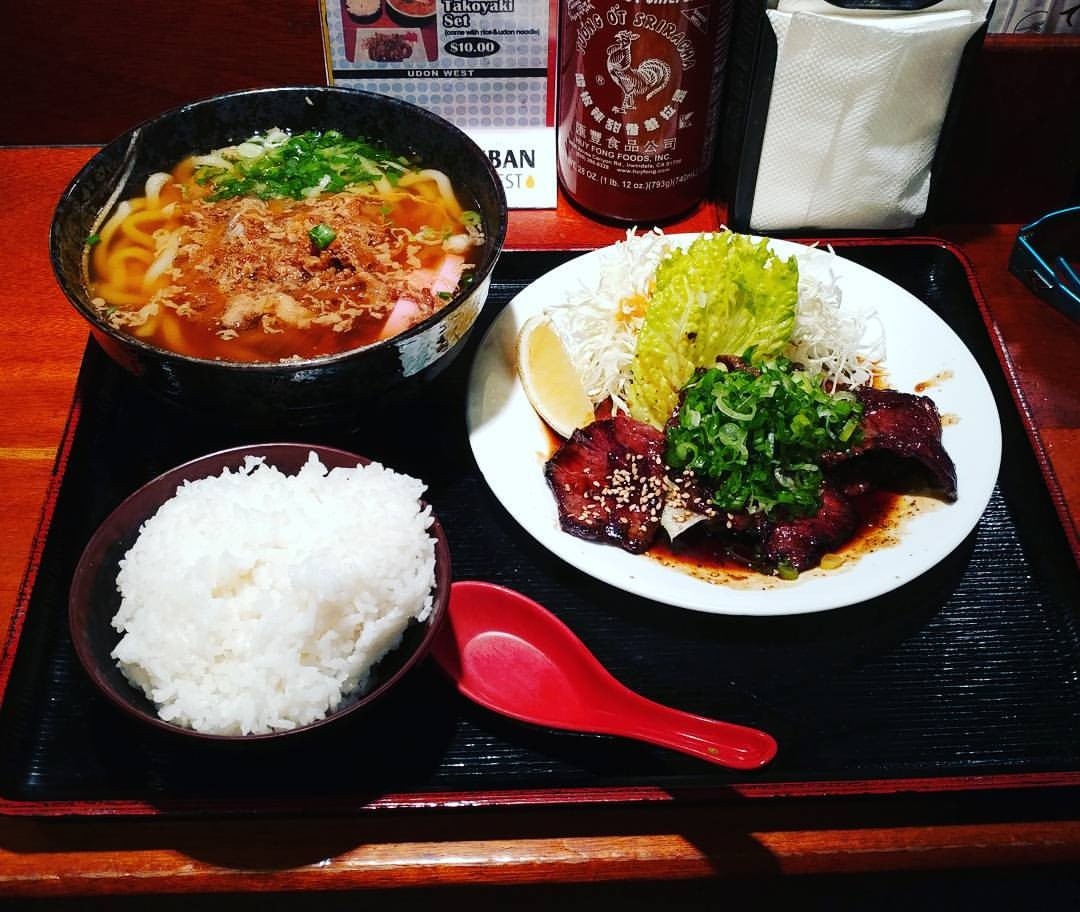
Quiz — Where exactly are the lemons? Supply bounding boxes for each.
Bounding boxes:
[515,309,598,440]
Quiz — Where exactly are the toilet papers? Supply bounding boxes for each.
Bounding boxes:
[754,0,983,240]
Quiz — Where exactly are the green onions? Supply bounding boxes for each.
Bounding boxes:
[666,342,866,522]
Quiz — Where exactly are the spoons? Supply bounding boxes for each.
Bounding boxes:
[436,581,778,772]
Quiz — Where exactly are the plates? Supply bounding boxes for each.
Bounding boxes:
[467,232,1001,618]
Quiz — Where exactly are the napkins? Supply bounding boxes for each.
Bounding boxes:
[748,6,972,232]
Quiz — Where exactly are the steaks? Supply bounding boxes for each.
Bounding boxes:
[544,381,956,576]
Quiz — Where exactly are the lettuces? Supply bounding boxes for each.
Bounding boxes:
[624,231,798,434]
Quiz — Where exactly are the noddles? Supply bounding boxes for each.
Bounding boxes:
[92,125,483,363]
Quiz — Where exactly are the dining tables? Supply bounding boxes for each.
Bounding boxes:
[1,147,1078,895]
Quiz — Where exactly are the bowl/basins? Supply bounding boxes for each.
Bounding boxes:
[50,85,510,413]
[66,445,449,743]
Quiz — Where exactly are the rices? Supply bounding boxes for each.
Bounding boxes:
[107,449,439,738]
